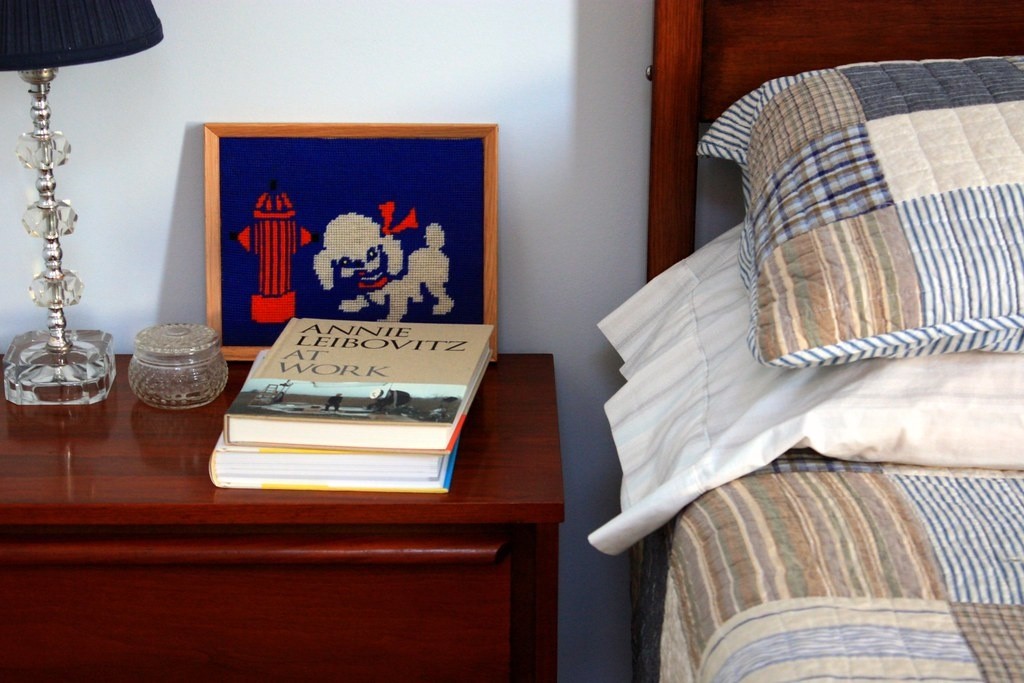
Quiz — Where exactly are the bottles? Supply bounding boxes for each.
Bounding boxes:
[128,323,229,410]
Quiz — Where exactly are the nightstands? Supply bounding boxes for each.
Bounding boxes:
[0,353,566,683]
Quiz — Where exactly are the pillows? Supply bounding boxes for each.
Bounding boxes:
[695,54,1024,370]
[587,222,1024,556]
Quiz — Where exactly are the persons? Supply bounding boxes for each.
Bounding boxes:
[322,392,343,412]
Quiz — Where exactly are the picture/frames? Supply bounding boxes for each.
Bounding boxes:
[204,122,500,362]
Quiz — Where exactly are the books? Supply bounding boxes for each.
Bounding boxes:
[207,311,494,493]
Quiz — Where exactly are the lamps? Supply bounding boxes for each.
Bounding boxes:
[0,0,164,406]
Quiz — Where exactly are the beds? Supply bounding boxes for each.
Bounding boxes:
[633,0,1024,683]
